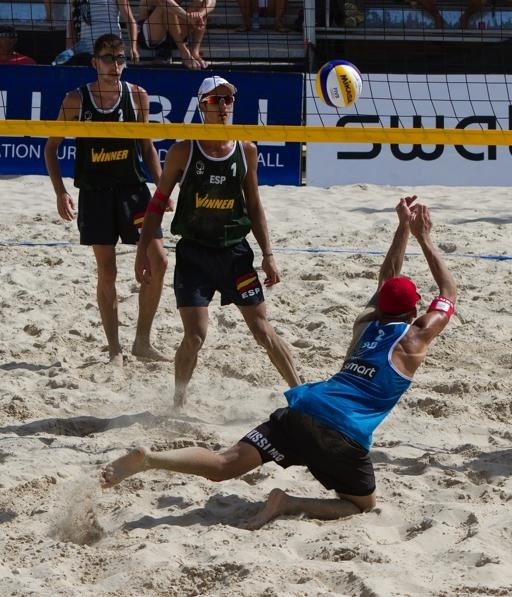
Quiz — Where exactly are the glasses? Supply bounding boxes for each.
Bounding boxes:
[201,95,235,106]
[94,53,127,64]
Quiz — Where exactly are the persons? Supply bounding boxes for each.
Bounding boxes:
[0,24,38,64]
[99,195,457,529]
[136,0,216,71]
[133,75,307,410]
[234,0,291,33]
[65,0,140,66]
[43,35,173,366]
[421,0,487,30]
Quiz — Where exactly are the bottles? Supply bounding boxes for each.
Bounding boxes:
[52,49,75,66]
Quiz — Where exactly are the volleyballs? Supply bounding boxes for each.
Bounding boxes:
[312,59,362,108]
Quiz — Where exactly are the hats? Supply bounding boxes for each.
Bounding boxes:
[198,76,238,96]
[377,277,421,312]
